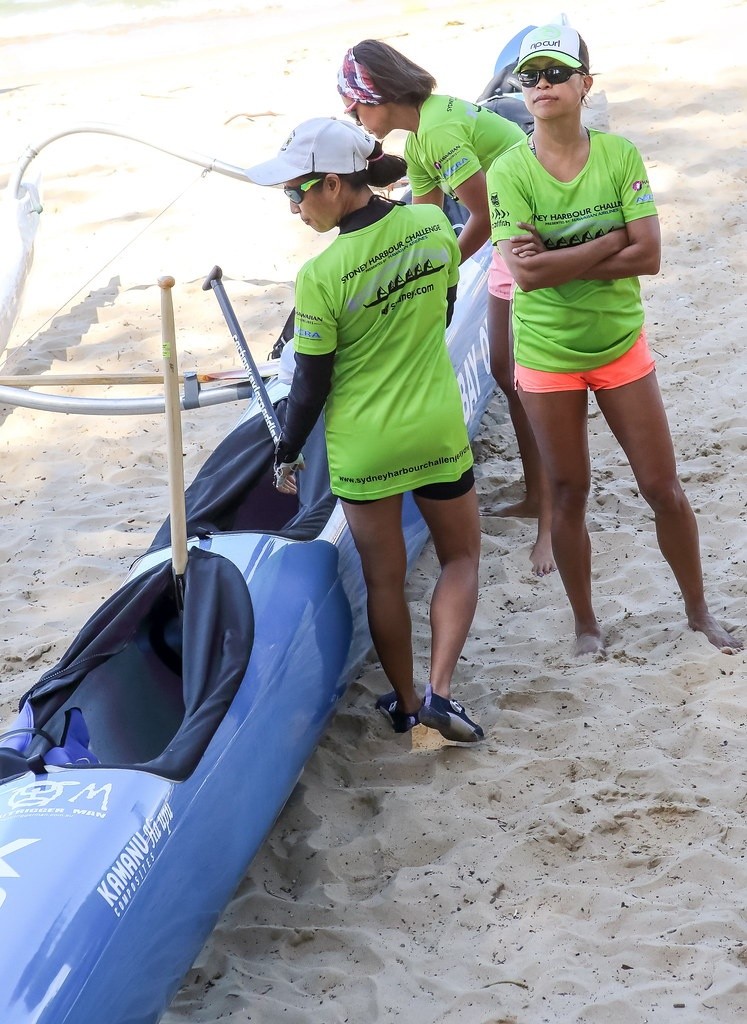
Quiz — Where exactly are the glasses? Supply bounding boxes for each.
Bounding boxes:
[284,179,321,204]
[518,66,586,88]
[344,100,359,120]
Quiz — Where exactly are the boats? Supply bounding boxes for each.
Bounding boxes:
[1,23,607,1024]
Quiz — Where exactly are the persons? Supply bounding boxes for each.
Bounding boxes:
[335,39,555,577]
[243,115,487,742]
[486,25,745,667]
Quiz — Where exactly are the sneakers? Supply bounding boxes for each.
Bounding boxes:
[418,683,484,742]
[374,690,423,733]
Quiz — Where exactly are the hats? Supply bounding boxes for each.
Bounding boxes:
[512,24,589,76]
[243,116,376,187]
